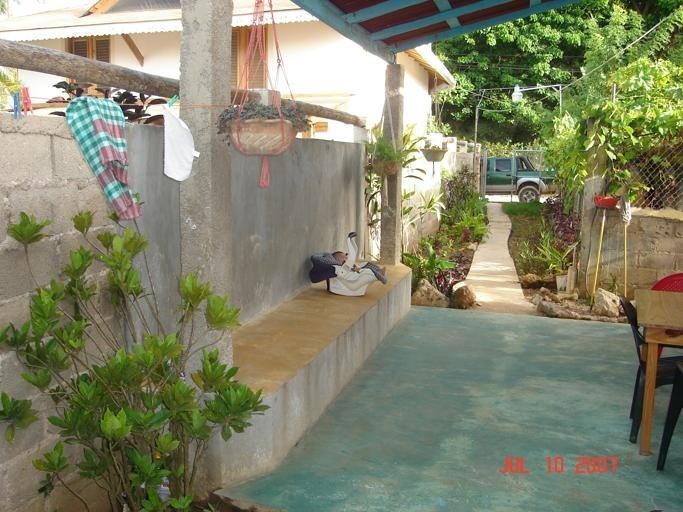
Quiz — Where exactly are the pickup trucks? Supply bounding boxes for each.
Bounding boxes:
[479,155,556,203]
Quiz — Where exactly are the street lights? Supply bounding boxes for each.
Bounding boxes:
[512,83,563,114]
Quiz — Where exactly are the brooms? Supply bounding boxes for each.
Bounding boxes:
[589,196,621,311]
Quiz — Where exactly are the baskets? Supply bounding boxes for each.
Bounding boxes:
[227,118,295,156]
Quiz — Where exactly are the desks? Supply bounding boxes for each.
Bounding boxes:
[633,288,683,457]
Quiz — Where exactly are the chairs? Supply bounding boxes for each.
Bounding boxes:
[617,295,683,471]
[652,273,683,359]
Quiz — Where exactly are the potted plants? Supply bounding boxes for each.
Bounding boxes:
[216,100,319,156]
[363,119,426,194]
[420,139,452,162]
